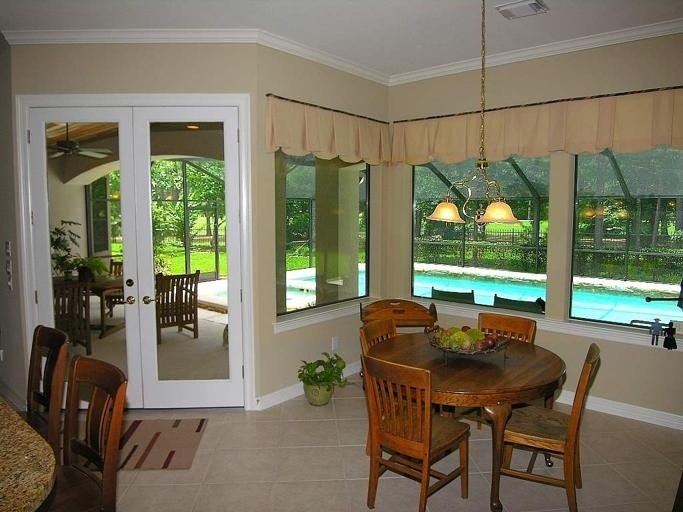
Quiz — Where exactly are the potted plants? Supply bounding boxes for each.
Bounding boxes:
[48,218,81,279]
[74,253,107,281]
[296,352,349,406]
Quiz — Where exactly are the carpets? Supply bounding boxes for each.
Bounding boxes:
[120,414,209,470]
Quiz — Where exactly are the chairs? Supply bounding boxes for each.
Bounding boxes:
[17,324,70,464]
[105,261,124,318]
[53,283,93,355]
[153,272,200,343]
[500,344,600,512]
[358,298,439,389]
[43,354,127,512]
[361,355,471,511]
[453,312,537,428]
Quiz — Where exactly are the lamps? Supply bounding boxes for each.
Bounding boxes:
[425,1,521,225]
